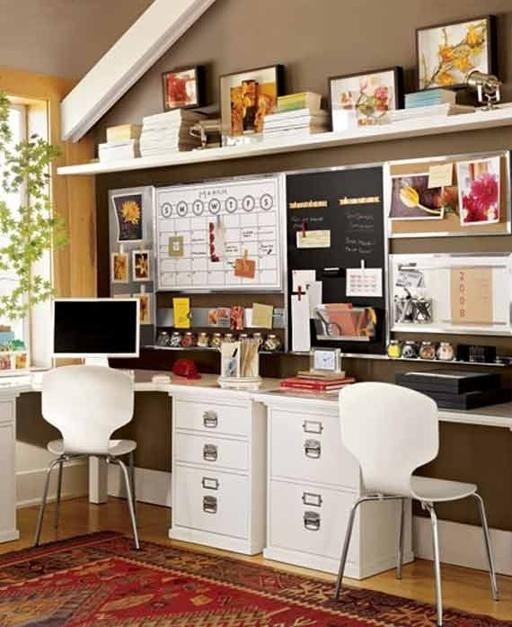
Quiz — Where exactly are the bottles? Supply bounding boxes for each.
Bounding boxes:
[387,339,454,361]
[157,331,283,352]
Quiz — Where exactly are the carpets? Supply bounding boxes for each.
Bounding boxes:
[0,531,512,627]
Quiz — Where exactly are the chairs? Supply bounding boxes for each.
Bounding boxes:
[34,365,141,551]
[334,381,499,626]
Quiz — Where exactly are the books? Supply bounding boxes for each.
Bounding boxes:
[261,88,329,146]
[279,371,355,396]
[140,110,205,158]
[386,89,473,124]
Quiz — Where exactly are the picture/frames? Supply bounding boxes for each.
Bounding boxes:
[416,15,498,89]
[328,67,404,131]
[219,65,285,133]
[162,65,207,112]
[283,162,389,360]
[386,151,511,239]
[154,172,283,294]
[389,252,512,337]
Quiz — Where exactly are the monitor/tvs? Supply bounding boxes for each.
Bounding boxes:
[52,298,141,366]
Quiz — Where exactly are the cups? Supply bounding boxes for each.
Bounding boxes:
[393,300,413,323]
[412,299,432,325]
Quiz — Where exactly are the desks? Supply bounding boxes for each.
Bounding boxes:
[255,389,512,581]
[0,369,279,556]
